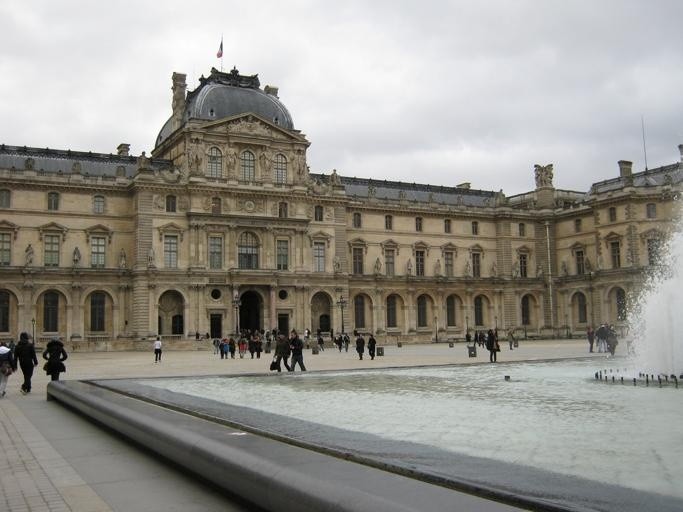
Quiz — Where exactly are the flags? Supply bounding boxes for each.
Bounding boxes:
[216,39,222,59]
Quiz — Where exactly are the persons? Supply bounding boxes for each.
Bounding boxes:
[486,328,498,363]
[23,243,156,270]
[354,333,364,360]
[10,331,38,394]
[6,339,18,372]
[331,254,594,282]
[366,334,376,360]
[209,325,349,360]
[506,327,514,350]
[0,339,12,397]
[152,335,161,363]
[472,328,500,348]
[586,321,619,355]
[137,151,147,169]
[288,330,306,371]
[330,169,337,185]
[272,330,290,372]
[42,336,69,381]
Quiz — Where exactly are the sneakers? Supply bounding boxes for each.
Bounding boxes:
[0,388,32,398]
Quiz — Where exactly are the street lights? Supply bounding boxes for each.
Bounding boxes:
[434,315,438,344]
[336,294,348,334]
[494,315,498,334]
[523,316,528,338]
[564,314,569,338]
[231,294,241,336]
[31,317,36,346]
[465,315,470,334]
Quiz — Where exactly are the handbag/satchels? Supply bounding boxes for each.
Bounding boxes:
[268,356,279,371]
[1,362,13,376]
